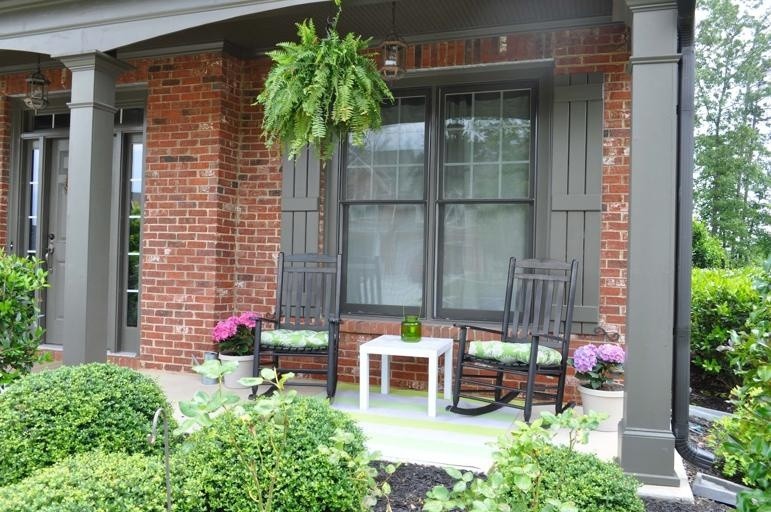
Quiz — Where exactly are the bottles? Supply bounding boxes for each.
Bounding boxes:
[401,313,422,343]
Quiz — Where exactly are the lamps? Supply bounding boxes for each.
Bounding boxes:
[23,54,51,116]
[379,1,408,88]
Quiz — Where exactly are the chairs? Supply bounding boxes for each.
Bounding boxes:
[446,255,579,434]
[249,252,344,405]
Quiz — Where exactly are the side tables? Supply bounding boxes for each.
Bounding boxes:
[359,335,453,420]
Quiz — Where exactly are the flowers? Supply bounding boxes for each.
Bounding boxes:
[567,343,626,390]
[211,312,262,354]
